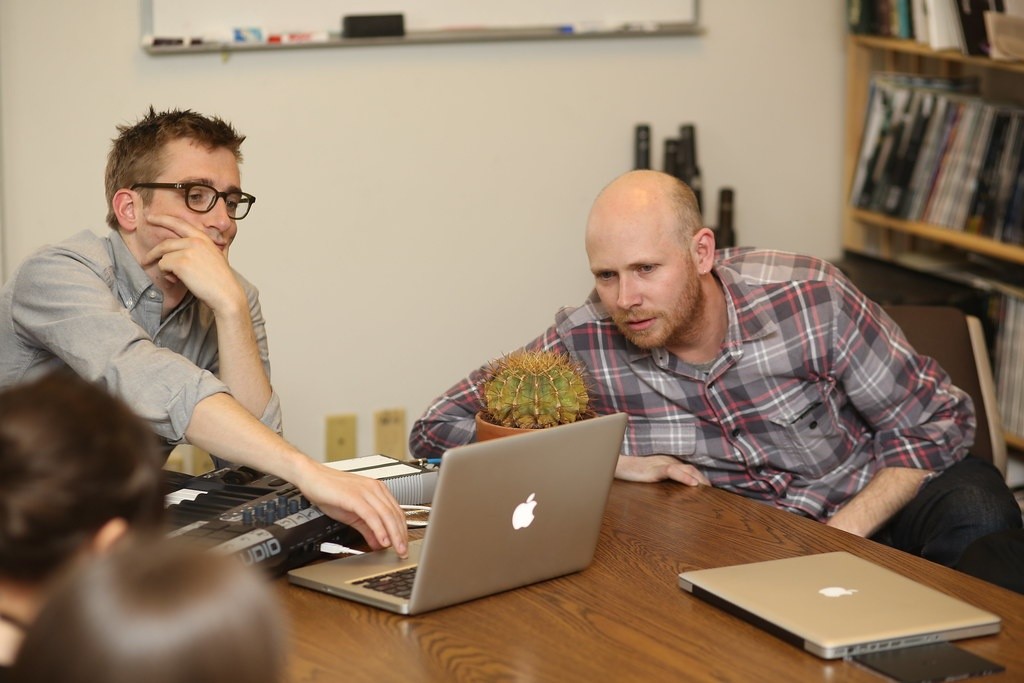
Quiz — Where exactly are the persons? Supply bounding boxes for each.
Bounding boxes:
[0,373,165,683]
[1,105,409,558]
[10,537,293,683]
[410,170,1024,592]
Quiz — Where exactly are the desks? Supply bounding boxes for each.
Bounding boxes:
[270,481,1024,683]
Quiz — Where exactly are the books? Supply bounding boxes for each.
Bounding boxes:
[634,124,733,248]
[846,0,1024,248]
[986,289,1024,437]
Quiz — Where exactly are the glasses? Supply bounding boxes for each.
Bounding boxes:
[132,182,257,220]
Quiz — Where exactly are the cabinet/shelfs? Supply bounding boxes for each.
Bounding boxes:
[838,0,1024,264]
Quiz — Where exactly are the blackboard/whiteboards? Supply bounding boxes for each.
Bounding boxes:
[140,0,707,55]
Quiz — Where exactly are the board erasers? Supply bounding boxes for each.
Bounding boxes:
[342,13,405,38]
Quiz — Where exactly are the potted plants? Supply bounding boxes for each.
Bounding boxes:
[476,350,599,443]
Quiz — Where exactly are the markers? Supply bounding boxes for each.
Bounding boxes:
[557,22,623,32]
[266,33,329,43]
[147,36,207,47]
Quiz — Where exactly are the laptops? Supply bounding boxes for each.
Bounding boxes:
[287,412,629,616]
[678,550,1002,660]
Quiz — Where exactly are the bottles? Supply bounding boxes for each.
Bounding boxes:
[635,124,649,170]
[713,189,736,249]
[665,125,701,214]
